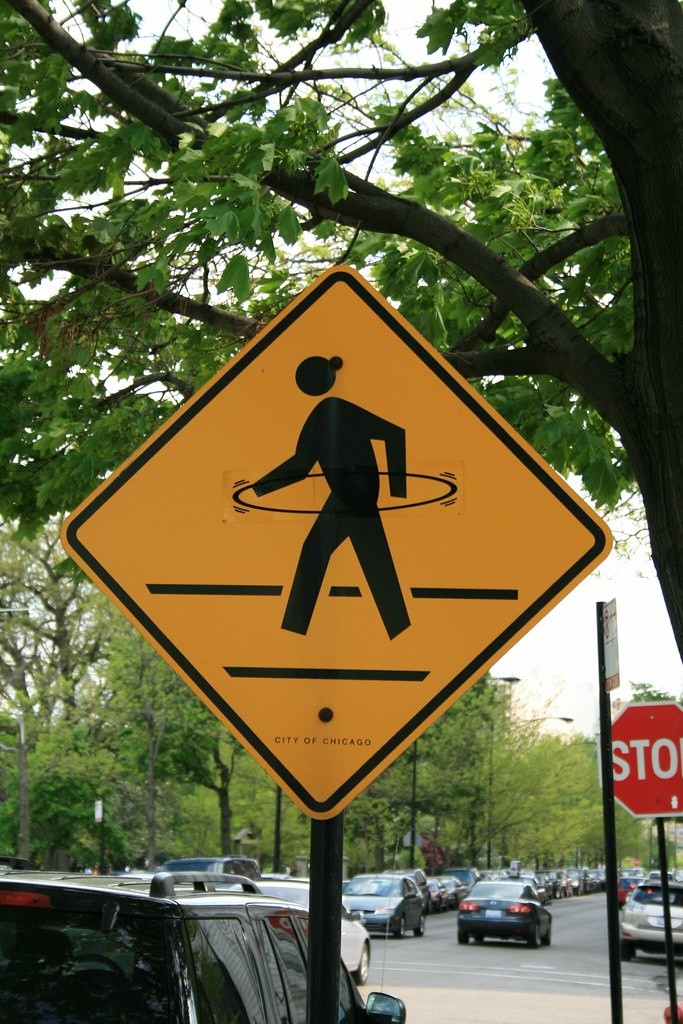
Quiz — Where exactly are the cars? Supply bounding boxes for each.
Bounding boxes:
[456,879,553,948]
[107,855,683,915]
[619,876,683,963]
[226,879,373,986]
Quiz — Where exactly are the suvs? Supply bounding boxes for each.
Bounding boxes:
[0,855,407,1023]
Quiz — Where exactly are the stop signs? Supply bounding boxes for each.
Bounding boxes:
[598,701,683,819]
